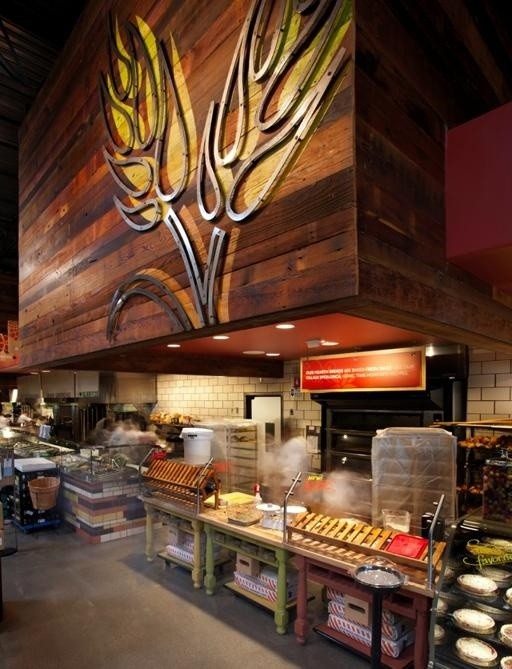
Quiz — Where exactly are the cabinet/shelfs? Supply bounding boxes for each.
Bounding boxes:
[371,428,457,539]
[183,419,258,494]
[432,419,512,535]
[429,518,512,669]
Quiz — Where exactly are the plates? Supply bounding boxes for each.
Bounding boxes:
[256,503,279,512]
[281,506,307,514]
[496,632,512,647]
[452,643,499,668]
[455,583,500,597]
[502,595,512,606]
[451,618,497,634]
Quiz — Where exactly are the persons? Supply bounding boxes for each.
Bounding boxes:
[81,408,159,460]
[0,411,56,438]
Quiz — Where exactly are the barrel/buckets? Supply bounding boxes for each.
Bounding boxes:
[179,428,213,464]
[28,478,60,508]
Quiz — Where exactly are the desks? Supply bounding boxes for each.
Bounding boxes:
[286,538,436,669]
[197,504,294,634]
[138,459,221,588]
